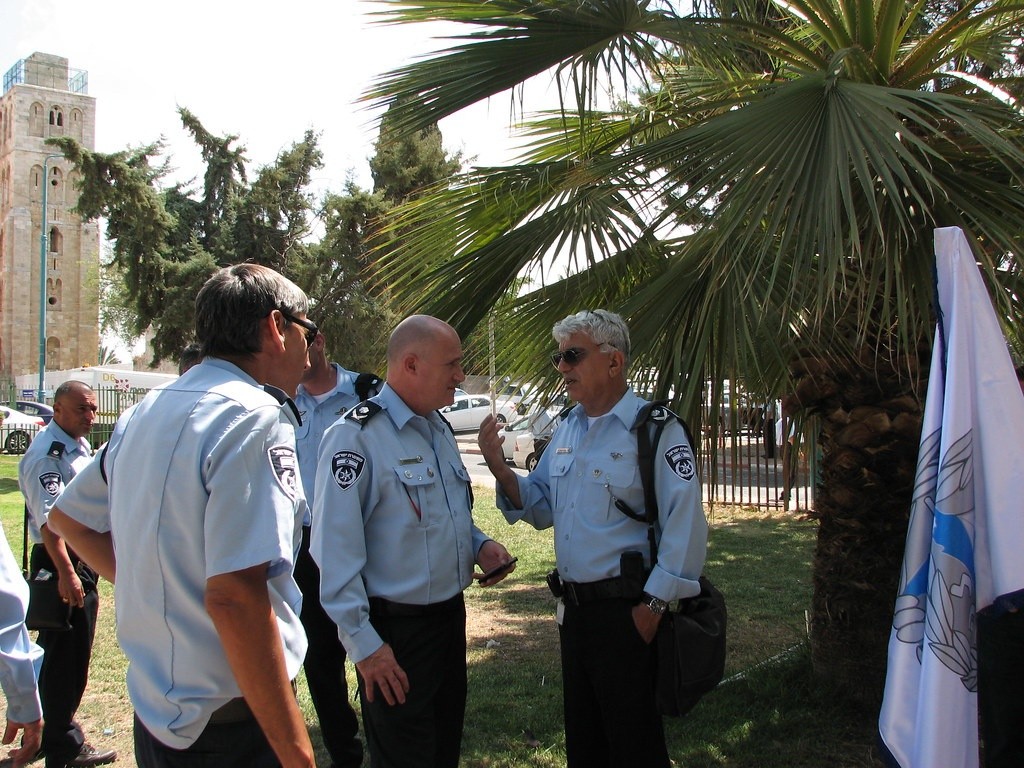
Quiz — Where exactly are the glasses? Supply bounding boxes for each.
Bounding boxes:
[264,309,318,348]
[550,341,617,370]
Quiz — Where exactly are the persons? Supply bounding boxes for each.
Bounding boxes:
[560,398,574,420]
[291,321,383,768]
[776,416,798,501]
[526,396,558,461]
[977,587,1024,768]
[762,400,778,458]
[307,314,515,767]
[478,309,710,767]
[0,522,45,768]
[21,380,118,768]
[45,262,318,767]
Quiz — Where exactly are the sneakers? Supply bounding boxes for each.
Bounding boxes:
[46,741,117,768]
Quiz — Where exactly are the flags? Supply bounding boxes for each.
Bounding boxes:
[879,226,1024,767]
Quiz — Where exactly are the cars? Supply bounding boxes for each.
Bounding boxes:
[0,401,54,454]
[438,367,764,474]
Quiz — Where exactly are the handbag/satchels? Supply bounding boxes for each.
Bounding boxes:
[654,575,727,718]
[26,580,72,631]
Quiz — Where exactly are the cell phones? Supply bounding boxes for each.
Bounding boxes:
[479,558,518,584]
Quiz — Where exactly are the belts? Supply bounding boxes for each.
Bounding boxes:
[560,569,652,606]
[369,592,463,616]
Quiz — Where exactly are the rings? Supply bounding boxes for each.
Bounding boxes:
[63,597,68,602]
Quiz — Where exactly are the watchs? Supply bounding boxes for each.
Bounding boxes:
[641,593,666,615]
